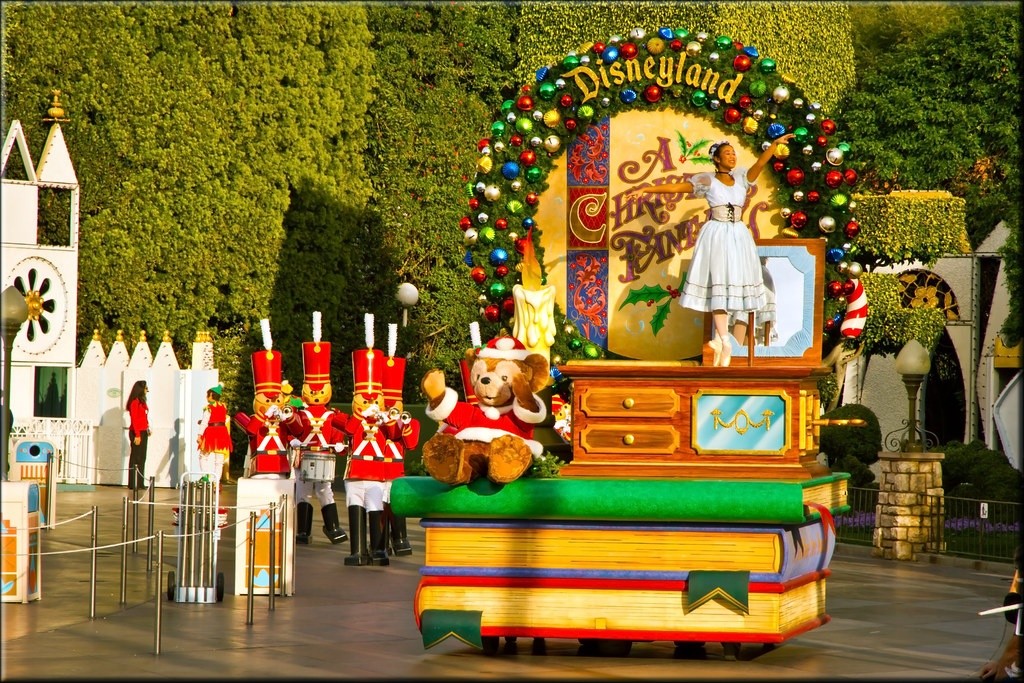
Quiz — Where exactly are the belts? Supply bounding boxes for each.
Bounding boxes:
[209,422,225,426]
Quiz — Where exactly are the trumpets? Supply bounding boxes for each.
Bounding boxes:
[274,406,294,418]
[372,408,412,425]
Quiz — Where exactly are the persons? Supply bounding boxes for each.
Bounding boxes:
[626,134,797,368]
[251,350,304,480]
[196,381,233,493]
[126,381,151,490]
[331,349,421,567]
[295,342,349,544]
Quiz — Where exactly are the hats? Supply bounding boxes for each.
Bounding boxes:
[210,384,223,396]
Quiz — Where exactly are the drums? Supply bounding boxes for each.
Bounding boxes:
[300,451,336,482]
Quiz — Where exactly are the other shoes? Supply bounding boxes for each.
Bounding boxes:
[138,485,148,490]
[708,339,721,367]
[721,340,732,368]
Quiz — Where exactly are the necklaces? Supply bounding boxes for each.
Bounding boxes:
[718,171,728,174]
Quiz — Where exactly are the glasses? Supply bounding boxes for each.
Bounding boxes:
[145,387,147,390]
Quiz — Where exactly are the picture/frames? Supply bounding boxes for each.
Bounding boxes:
[701,237,826,365]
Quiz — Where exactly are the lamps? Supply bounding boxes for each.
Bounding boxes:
[895,339,930,376]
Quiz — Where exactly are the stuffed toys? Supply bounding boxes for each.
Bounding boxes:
[421,336,549,483]
